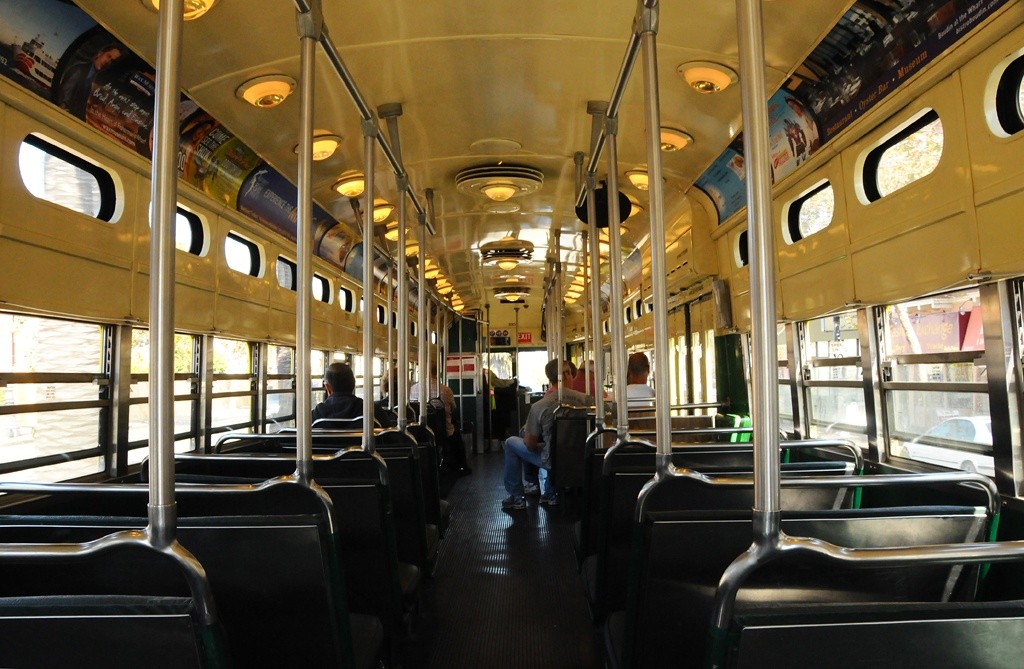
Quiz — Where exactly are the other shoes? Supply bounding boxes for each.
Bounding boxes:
[502,494,526,510]
[522,482,540,494]
[540,492,559,505]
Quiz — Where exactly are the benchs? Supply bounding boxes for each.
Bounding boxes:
[545,400,1024,669]
[0,397,457,669]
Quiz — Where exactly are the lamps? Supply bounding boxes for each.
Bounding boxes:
[140,0,466,316]
[481,249,533,272]
[562,60,741,307]
[453,164,546,202]
[493,287,532,302]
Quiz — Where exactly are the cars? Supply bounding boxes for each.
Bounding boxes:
[897,415,996,475]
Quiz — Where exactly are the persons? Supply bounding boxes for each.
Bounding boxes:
[604,352,655,406]
[177,120,215,180]
[851,55,901,108]
[784,95,820,155]
[431,365,472,473]
[867,20,888,45]
[311,363,392,430]
[483,368,514,436]
[374,367,440,447]
[501,359,611,509]
[827,79,849,104]
[539,361,577,503]
[573,360,610,398]
[59,42,124,123]
[146,101,199,160]
